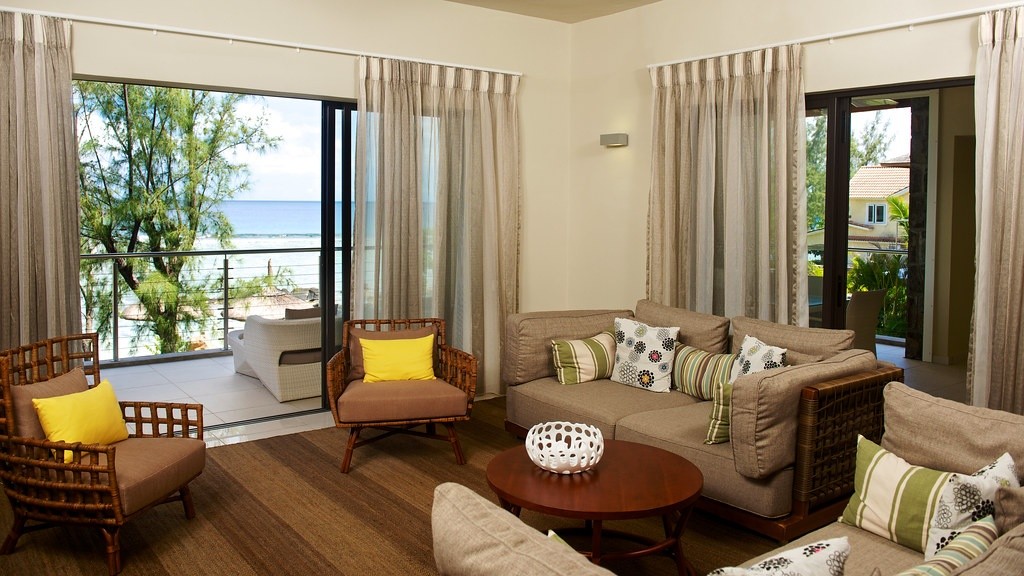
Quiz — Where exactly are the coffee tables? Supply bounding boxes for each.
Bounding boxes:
[478,439,704,576]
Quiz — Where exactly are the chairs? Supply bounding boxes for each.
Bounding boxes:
[318,320,476,474]
[809,287,889,359]
[0,334,207,576]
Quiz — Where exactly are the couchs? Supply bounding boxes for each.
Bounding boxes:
[498,303,904,544]
[243,317,345,405]
[429,383,1024,576]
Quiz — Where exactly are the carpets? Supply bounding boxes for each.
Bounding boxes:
[0,393,783,576]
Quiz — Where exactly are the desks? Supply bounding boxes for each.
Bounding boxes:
[228,328,261,379]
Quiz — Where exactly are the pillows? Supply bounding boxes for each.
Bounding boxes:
[549,317,614,386]
[282,304,337,364]
[606,316,680,391]
[733,334,786,388]
[350,323,439,382]
[666,341,733,401]
[705,383,745,445]
[704,435,1024,576]
[14,366,128,456]
